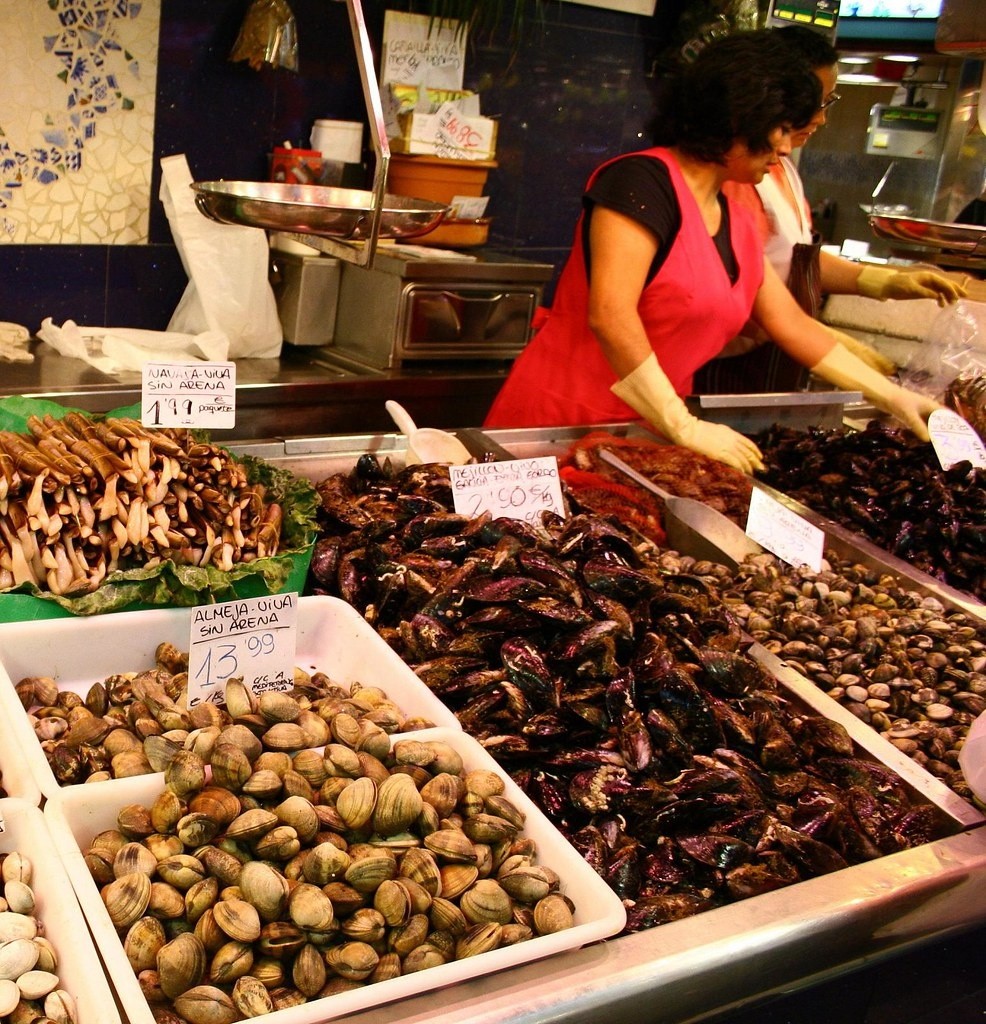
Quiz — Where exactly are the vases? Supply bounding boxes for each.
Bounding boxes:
[386,153,501,246]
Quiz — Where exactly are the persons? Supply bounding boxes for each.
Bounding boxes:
[481,36,950,475]
[692,24,968,394]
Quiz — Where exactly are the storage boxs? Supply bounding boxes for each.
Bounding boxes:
[42,726,627,1023]
[0,424,318,624]
[385,114,498,161]
[0,591,462,796]
[0,798,121,1024]
[0,690,41,806]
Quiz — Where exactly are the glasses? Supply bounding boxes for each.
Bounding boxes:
[817,93,841,111]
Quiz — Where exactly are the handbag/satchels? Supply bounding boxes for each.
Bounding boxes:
[157,153,284,358]
[35,318,231,383]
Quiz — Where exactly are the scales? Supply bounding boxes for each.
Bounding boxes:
[187,0,449,267]
[866,160,985,269]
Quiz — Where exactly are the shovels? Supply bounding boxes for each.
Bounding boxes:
[595,445,765,568]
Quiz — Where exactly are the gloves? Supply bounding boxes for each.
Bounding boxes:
[857,265,967,309]
[810,343,949,443]
[810,317,897,376]
[609,350,765,476]
